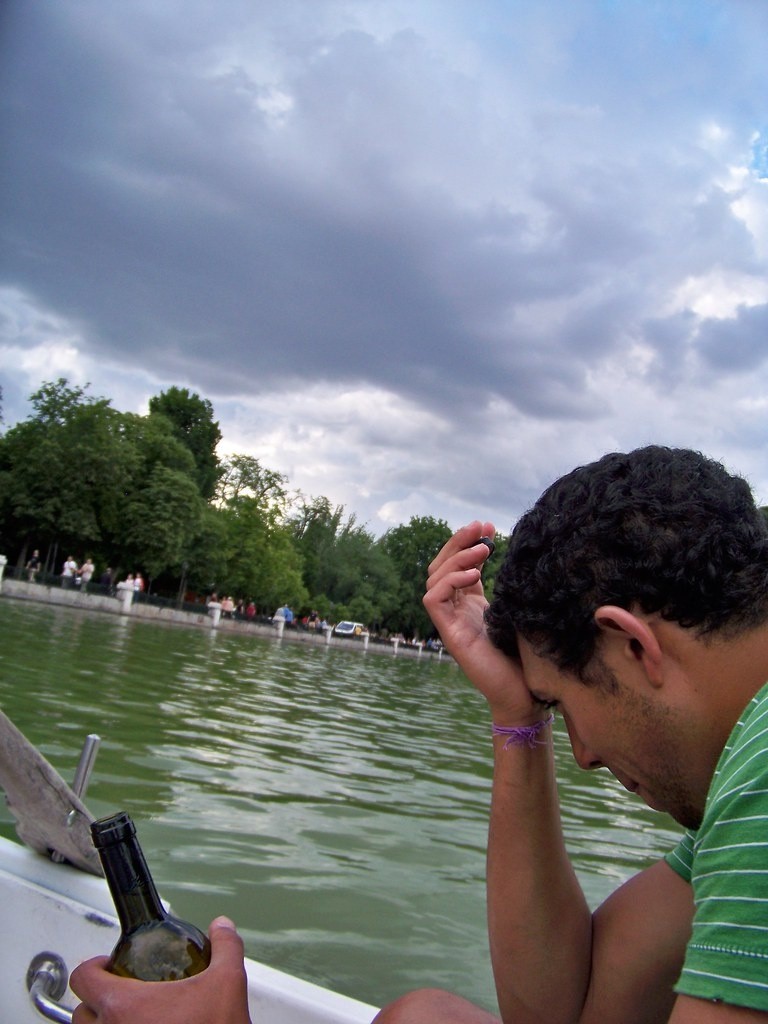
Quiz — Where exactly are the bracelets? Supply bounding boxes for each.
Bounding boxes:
[492,715,554,748]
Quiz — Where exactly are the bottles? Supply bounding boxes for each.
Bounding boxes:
[89,810,212,983]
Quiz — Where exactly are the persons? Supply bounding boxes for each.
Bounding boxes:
[25,549,40,583]
[98,567,112,593]
[68,445,768,1024]
[283,603,330,634]
[209,592,255,621]
[64,556,95,592]
[355,625,361,639]
[402,636,444,652]
[126,573,144,603]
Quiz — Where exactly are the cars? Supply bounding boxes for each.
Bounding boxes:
[335,620,364,637]
